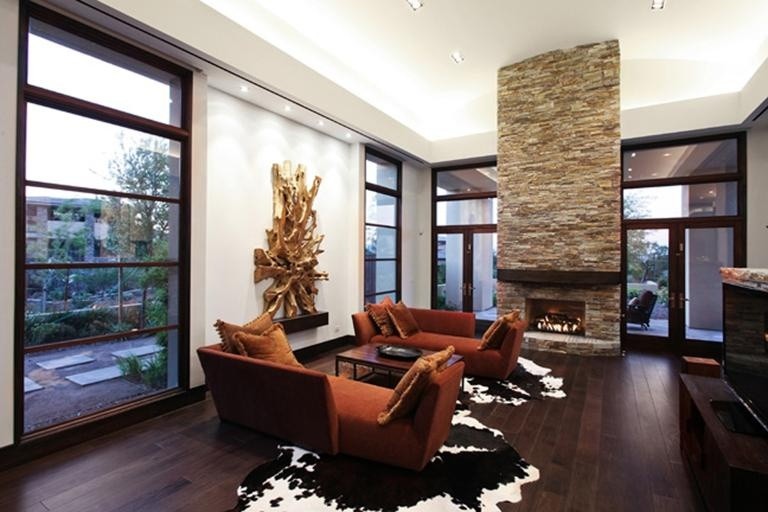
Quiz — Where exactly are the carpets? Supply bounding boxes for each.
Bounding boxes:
[227,356,568,512]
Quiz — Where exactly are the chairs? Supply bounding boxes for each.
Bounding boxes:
[625,291,657,330]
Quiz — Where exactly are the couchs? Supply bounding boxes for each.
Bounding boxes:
[352,308,528,381]
[197,343,465,472]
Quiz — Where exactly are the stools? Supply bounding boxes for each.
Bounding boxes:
[682,356,720,378]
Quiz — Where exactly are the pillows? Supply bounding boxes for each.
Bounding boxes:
[214,311,273,355]
[640,291,652,309]
[476,308,521,351]
[385,301,420,339]
[377,346,455,426]
[233,323,303,368]
[365,295,394,336]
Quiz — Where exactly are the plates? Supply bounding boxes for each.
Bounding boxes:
[378,344,422,361]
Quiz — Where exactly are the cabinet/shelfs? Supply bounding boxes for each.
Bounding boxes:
[680,374,768,512]
